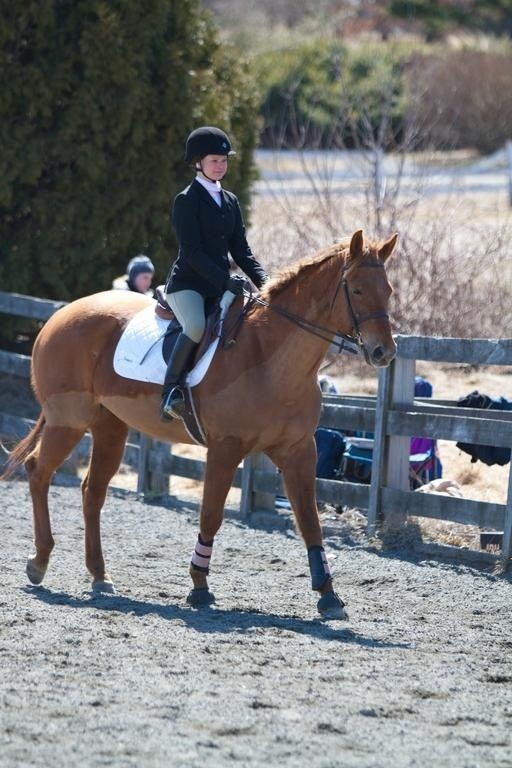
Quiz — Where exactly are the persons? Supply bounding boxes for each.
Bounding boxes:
[410,376,442,490]
[112,255,155,298]
[159,126,270,424]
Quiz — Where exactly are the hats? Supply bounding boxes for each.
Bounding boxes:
[185,126,237,165]
[127,255,155,285]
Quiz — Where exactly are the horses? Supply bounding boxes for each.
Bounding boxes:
[0,229,398,614]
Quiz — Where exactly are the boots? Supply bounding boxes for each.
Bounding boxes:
[160,330,201,424]
